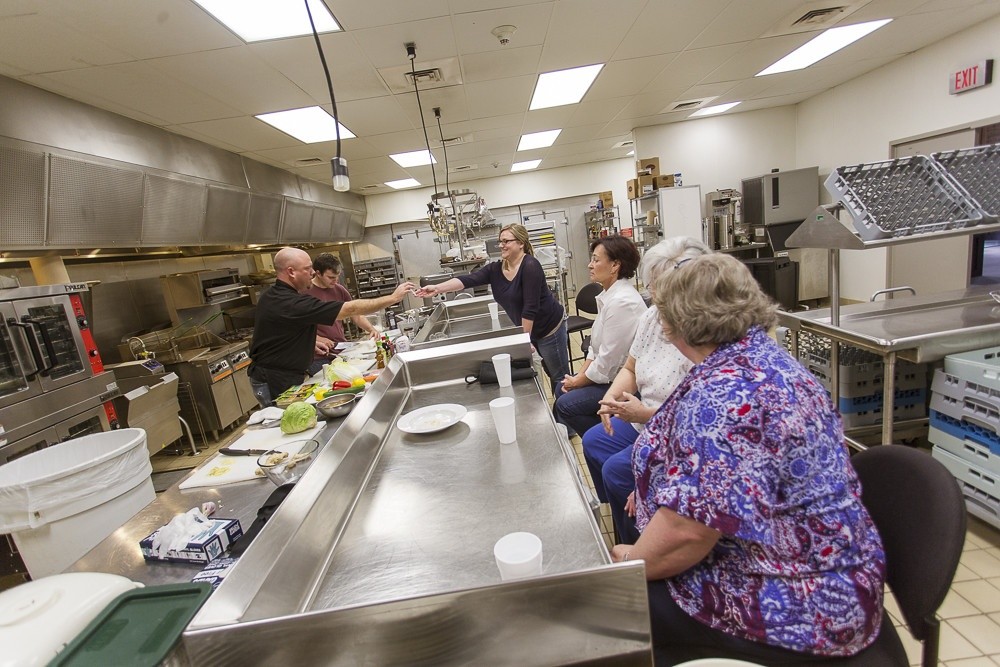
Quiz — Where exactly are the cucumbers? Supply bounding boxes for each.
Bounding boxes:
[323,385,365,397]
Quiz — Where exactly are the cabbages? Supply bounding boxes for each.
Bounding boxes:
[281,401,318,433]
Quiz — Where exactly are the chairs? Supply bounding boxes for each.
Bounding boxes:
[563,283,604,375]
[840,446,965,667]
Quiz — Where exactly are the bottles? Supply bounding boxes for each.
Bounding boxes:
[386,306,398,329]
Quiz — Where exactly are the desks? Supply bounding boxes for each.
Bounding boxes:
[183,327,654,667]
[412,294,523,352]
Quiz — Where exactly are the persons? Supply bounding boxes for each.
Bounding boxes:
[606,250,888,667]
[247,244,415,408]
[550,234,649,443]
[581,235,718,552]
[413,222,571,401]
[304,253,380,369]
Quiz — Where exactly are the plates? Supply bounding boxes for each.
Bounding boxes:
[397,404,467,434]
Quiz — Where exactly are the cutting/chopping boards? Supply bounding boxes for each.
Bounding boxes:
[178,420,327,491]
[305,358,377,384]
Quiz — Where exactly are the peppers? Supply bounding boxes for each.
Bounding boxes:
[332,381,352,390]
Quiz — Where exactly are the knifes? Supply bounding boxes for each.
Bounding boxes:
[220,448,282,458]
[333,342,360,350]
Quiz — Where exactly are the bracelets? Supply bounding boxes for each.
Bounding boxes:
[621,552,630,563]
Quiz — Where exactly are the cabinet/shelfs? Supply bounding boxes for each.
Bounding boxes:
[580,205,620,261]
[628,185,705,255]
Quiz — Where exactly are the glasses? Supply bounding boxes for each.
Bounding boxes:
[498,238,517,243]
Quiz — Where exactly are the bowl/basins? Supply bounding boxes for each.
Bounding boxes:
[258,439,321,486]
[316,392,356,415]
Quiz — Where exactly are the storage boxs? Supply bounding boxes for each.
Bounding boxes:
[627,178,639,199]
[653,175,675,188]
[636,157,660,176]
[638,173,654,198]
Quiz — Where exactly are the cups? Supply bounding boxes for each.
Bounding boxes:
[489,397,516,443]
[488,302,499,319]
[492,354,513,386]
